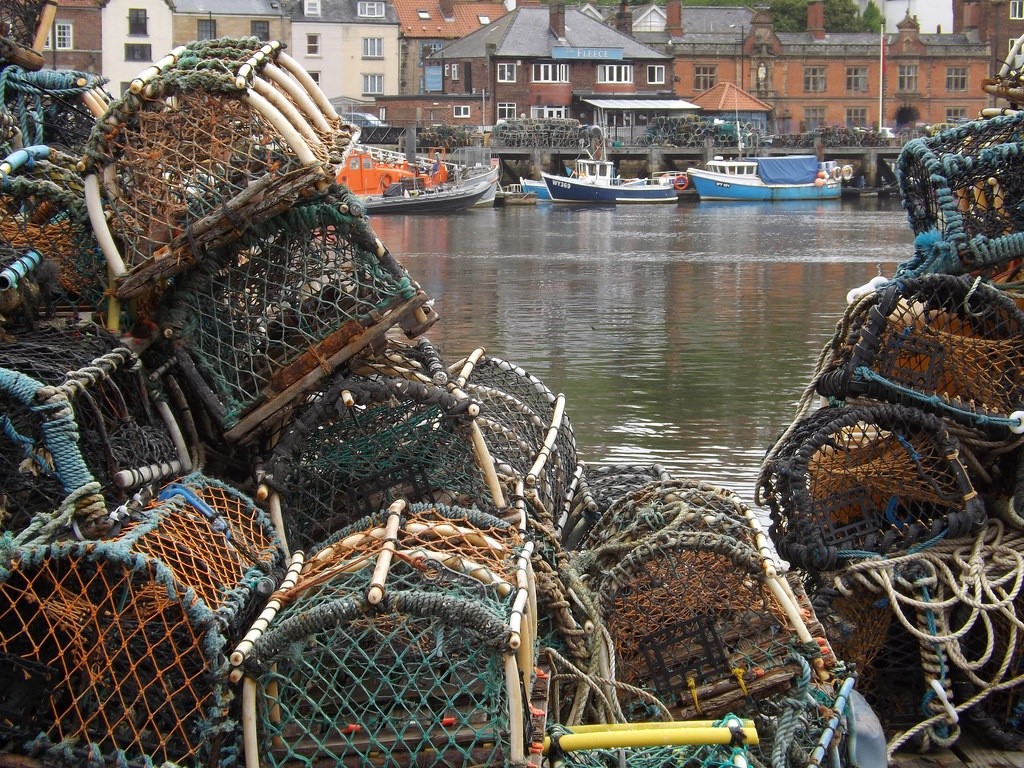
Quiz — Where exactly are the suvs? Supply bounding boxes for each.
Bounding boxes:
[882,127,898,137]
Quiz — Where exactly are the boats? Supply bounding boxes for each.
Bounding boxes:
[350,181,491,214]
[540,104,688,204]
[519,175,647,201]
[687,88,853,200]
[337,121,501,208]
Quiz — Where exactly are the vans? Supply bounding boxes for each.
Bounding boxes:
[342,113,385,126]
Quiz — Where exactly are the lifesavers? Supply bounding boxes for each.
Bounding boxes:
[674,175,689,190]
[379,173,392,188]
[832,166,842,181]
[842,165,853,181]
